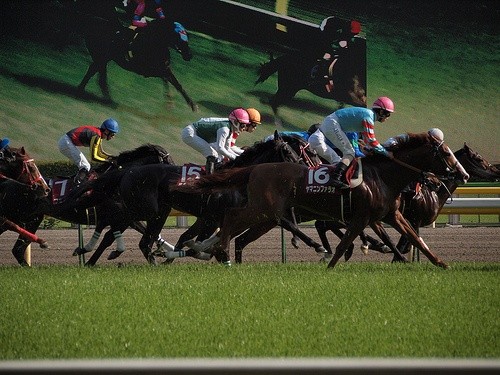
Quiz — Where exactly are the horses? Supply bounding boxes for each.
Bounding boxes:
[12,143,176,271]
[69,12,199,114]
[70,130,500,284]
[0,145,52,249]
[254,38,367,126]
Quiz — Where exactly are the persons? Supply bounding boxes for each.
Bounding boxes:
[181,108,252,177]
[58,119,119,198]
[114,0,165,59]
[221,107,262,162]
[264,123,320,143]
[315,15,361,92]
[308,125,366,166]
[318,96,395,188]
[383,125,445,149]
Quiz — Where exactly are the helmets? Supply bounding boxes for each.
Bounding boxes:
[247,109,263,124]
[101,117,121,133]
[429,127,444,144]
[229,108,250,124]
[373,96,394,113]
